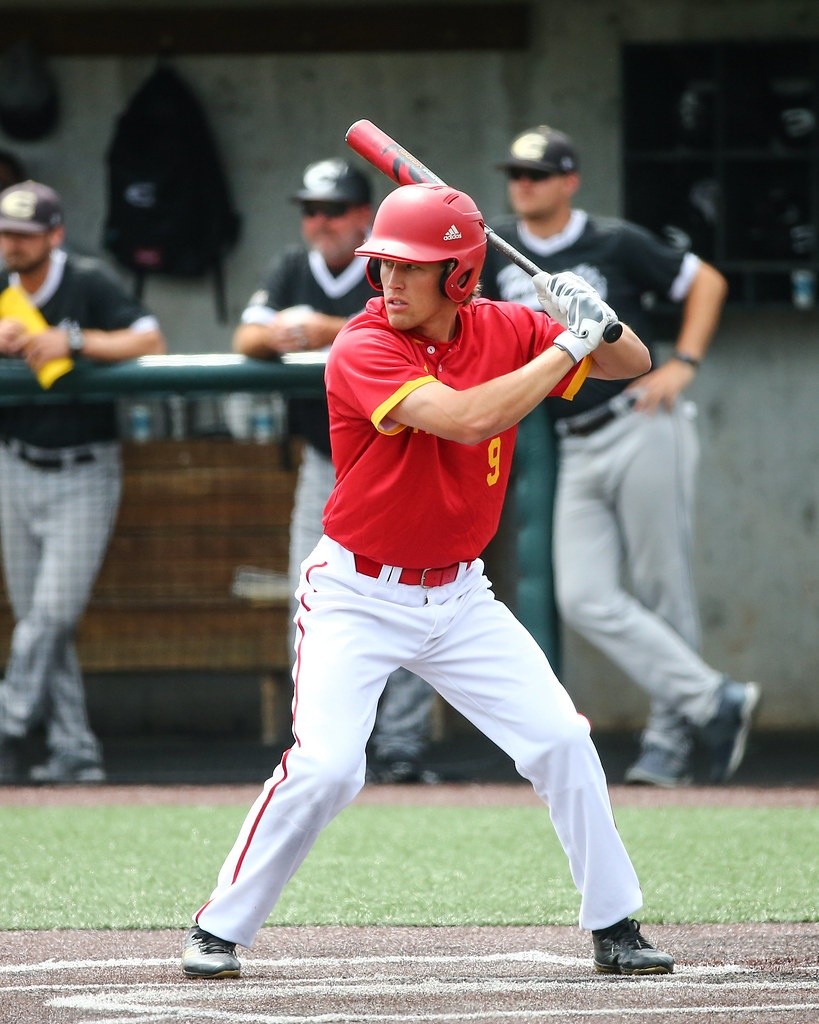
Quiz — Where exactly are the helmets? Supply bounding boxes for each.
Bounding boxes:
[353,183,486,302]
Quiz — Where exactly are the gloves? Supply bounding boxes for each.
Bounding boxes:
[531,270,601,330]
[553,293,619,364]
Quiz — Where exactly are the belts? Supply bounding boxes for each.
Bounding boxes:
[5,441,96,471]
[353,552,474,589]
[567,397,637,437]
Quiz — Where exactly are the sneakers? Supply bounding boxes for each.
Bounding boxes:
[591,917,673,975]
[181,925,241,980]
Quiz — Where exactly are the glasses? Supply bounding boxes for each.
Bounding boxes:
[505,166,554,182]
[298,202,355,217]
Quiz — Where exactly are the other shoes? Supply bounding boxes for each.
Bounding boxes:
[697,675,759,785]
[626,746,695,788]
[28,751,106,784]
[0,735,30,786]
[366,751,421,788]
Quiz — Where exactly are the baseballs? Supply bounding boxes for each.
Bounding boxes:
[343,118,624,345]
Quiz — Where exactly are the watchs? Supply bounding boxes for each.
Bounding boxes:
[69,326,84,362]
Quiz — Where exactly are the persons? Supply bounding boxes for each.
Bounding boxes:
[182,183,678,978]
[475,128,763,790]
[1,179,167,786]
[232,157,449,788]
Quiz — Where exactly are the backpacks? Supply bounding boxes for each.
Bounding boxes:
[100,69,246,283]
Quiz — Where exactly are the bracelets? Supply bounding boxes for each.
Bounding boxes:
[671,350,699,370]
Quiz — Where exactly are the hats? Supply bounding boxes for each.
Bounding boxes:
[289,159,371,205]
[0,181,62,239]
[494,125,580,174]
